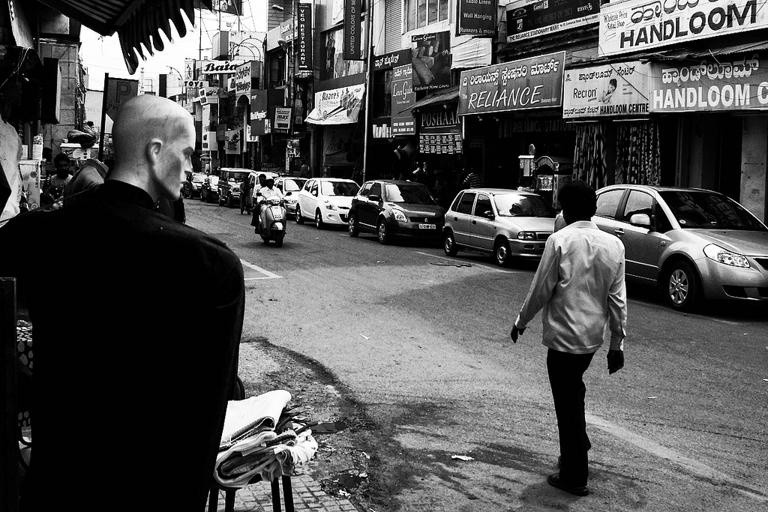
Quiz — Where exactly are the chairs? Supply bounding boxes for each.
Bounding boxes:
[207,377,294,512]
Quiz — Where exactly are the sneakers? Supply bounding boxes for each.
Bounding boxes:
[546,470,589,496]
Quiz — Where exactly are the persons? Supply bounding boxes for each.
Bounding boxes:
[252,173,267,203]
[61,158,112,204]
[511,177,627,496]
[54,153,75,186]
[0,94,246,512]
[251,177,290,234]
[602,80,617,103]
[462,165,481,188]
